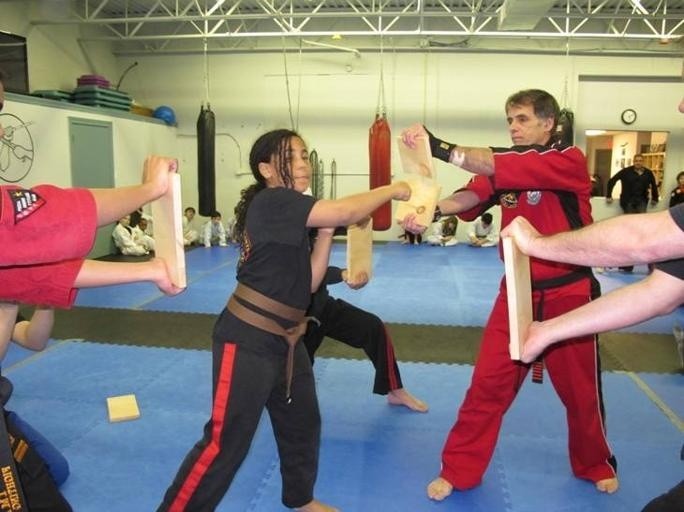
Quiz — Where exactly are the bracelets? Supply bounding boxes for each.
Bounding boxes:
[423,125,456,162]
[432,206,441,222]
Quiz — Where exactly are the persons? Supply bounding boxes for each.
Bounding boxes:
[427,213,499,247]
[156,129,412,512]
[304,215,429,412]
[0,303,69,486]
[607,154,658,271]
[0,82,187,512]
[500,59,684,512]
[397,89,619,501]
[112,208,238,256]
[670,172,684,207]
